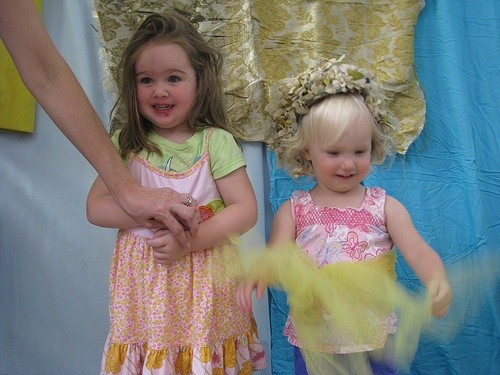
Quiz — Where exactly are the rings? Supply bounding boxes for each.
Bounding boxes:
[184,195,193,206]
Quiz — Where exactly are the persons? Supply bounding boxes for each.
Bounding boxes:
[86,15,266,375]
[235,60,452,375]
[0,0,202,250]
